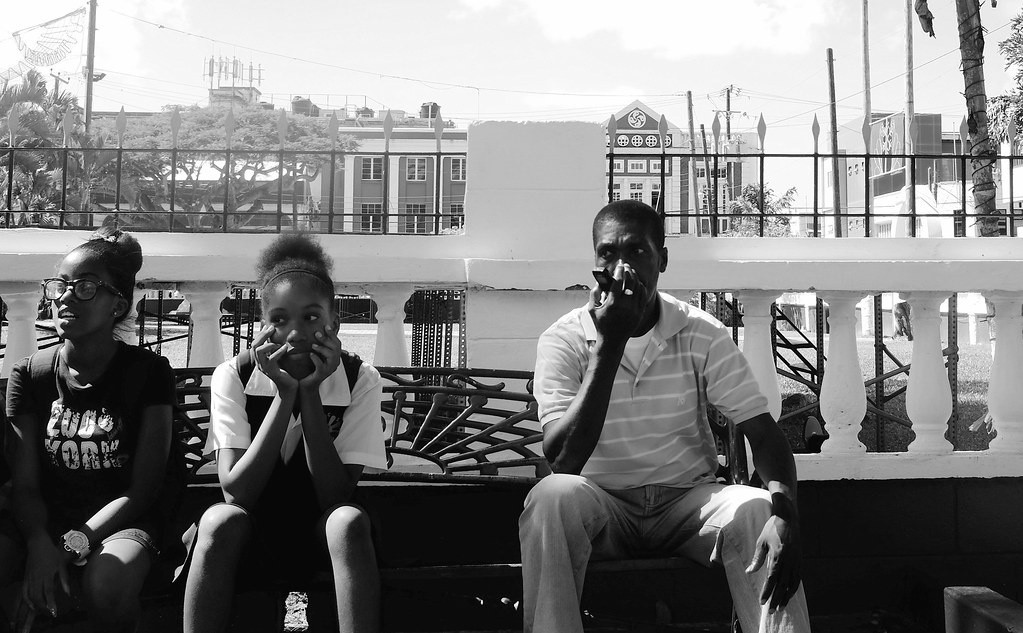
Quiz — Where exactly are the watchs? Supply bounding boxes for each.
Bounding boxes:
[60,528,90,566]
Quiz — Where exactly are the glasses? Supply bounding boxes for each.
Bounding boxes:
[41,278,124,301]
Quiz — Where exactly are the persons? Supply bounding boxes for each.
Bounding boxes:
[518,200,810,633]
[185,235,388,633]
[1,225,176,633]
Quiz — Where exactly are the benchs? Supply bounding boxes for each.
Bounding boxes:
[1,366,750,633]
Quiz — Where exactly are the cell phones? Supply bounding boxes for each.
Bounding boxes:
[593,267,613,296]
